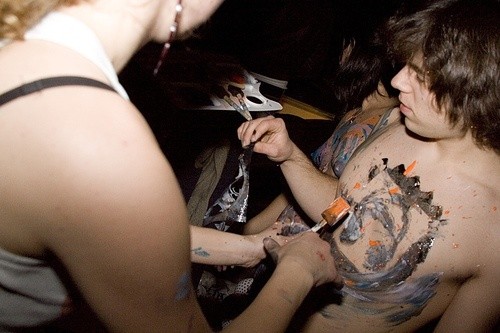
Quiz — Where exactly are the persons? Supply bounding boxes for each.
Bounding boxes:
[1,0,346,333]
[211,0,500,333]
